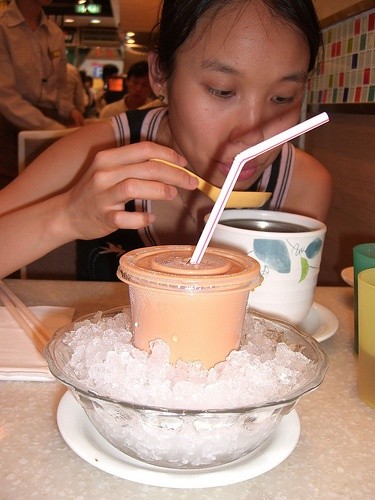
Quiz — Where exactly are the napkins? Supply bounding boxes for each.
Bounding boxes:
[0,305,76,383]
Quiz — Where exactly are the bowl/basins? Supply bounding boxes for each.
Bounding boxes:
[44,306,330,469]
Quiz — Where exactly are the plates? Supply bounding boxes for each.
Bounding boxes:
[341,266,354,287]
[56,390,301,488]
[296,301,339,343]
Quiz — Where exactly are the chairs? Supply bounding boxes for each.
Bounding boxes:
[16,127,79,281]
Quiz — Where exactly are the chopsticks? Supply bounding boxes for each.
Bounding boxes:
[0,280,52,355]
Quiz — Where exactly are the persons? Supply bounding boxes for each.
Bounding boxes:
[65,63,88,112]
[0,0,84,189]
[96,64,123,112]
[99,62,155,117]
[0,0,332,281]
[80,71,97,117]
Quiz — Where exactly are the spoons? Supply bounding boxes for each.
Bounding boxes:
[150,158,272,209]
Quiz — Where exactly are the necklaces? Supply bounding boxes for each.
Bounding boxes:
[177,186,198,225]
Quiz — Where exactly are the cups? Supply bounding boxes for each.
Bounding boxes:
[117,246,264,371]
[357,268,375,410]
[353,243,375,354]
[205,209,327,326]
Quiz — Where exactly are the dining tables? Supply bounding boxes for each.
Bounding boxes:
[0,281,375,500]
